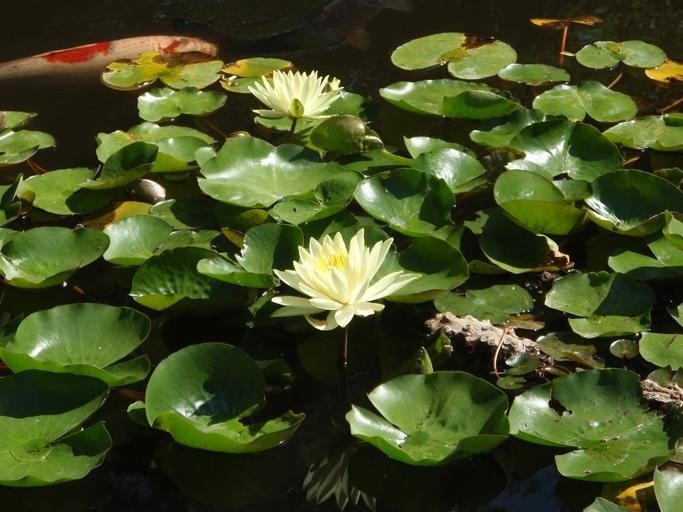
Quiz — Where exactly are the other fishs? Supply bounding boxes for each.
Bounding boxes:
[0,36,217,81]
